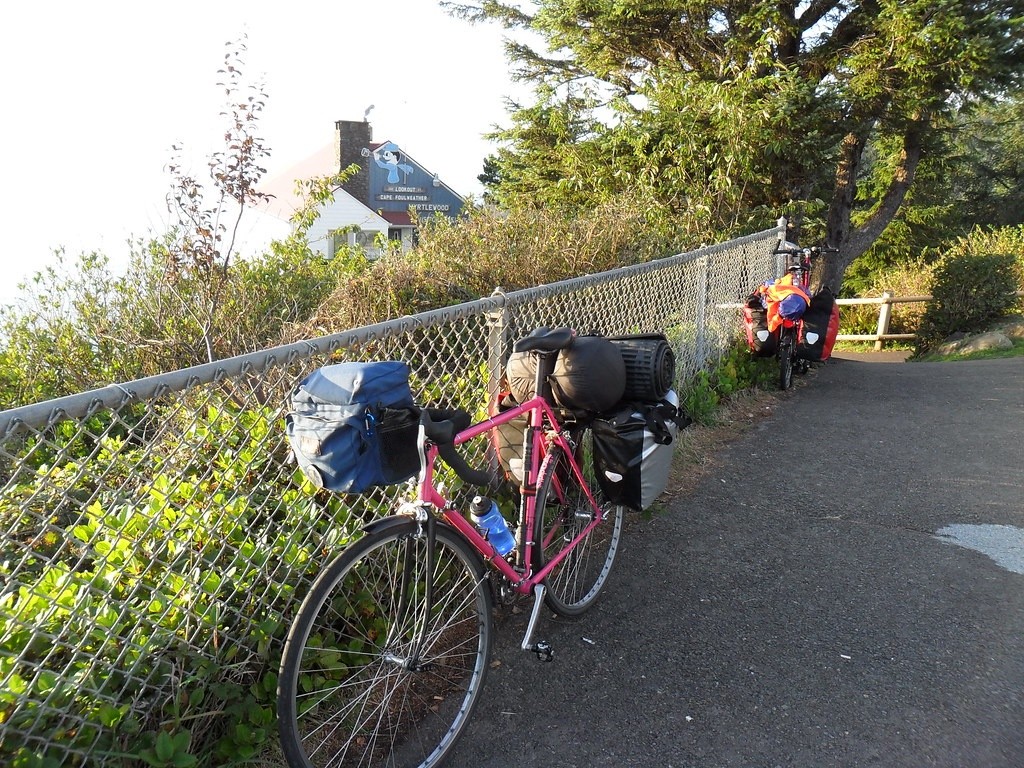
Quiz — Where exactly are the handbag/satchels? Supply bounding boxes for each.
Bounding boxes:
[283,357,420,492]
[491,396,530,489]
[590,391,683,511]
[799,290,840,363]
[745,291,780,357]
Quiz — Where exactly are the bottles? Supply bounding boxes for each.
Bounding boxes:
[469,496,516,555]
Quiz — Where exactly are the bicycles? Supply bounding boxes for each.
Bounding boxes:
[772,238,840,390]
[275,321,626,768]
